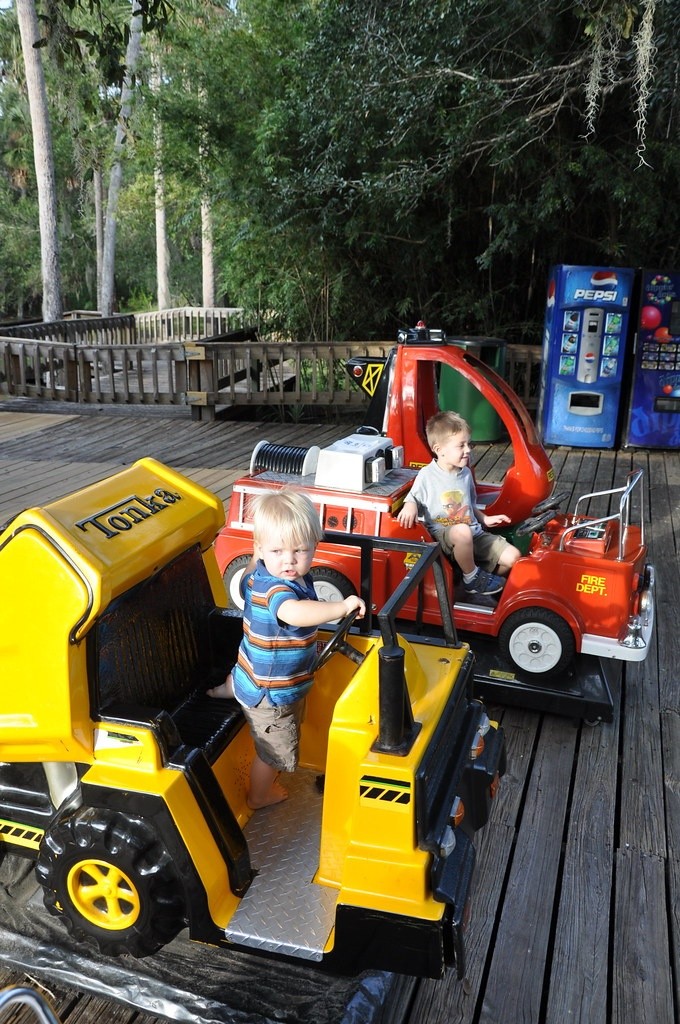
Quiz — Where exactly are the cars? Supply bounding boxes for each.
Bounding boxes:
[0,457,508,982]
[206,337,657,728]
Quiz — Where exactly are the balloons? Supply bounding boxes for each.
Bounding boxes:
[655,327,672,342]
[642,306,661,330]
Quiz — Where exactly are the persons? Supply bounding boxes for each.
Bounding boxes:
[207,490,365,810]
[397,410,521,595]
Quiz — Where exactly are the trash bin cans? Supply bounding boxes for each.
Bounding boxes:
[438,335,507,446]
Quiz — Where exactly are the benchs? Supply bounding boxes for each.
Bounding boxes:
[83,543,253,896]
[386,508,462,608]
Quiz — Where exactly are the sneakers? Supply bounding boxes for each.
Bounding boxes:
[464,566,506,595]
